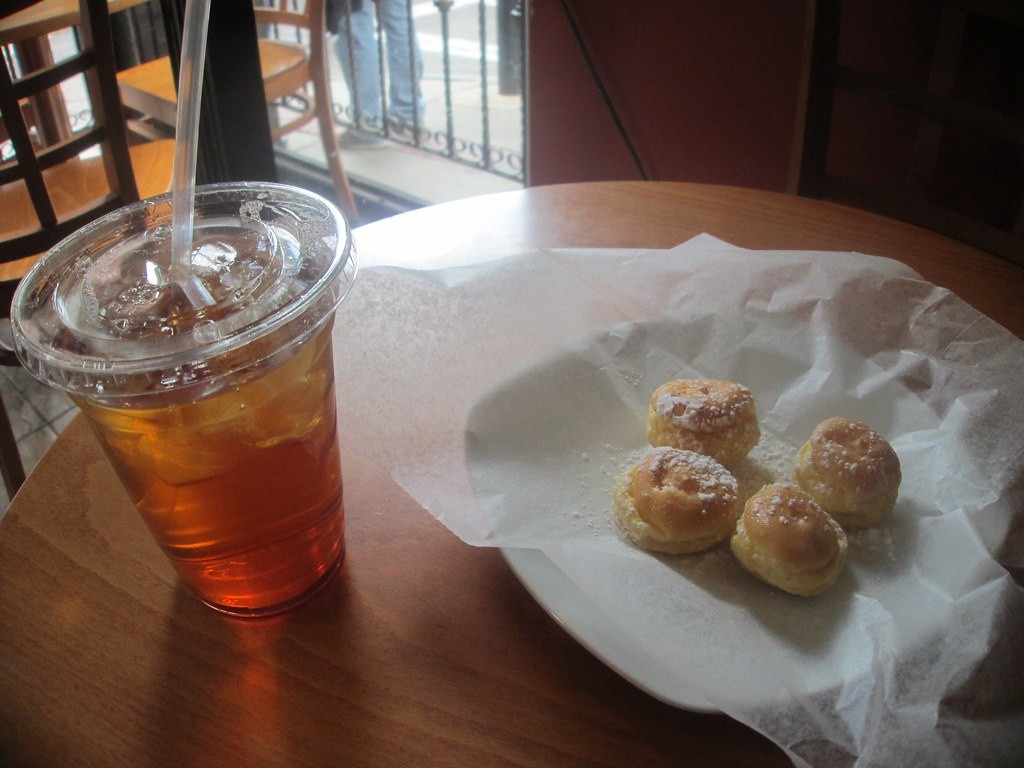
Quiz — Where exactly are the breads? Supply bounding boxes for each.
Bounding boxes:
[645,378,761,468]
[794,417,902,531]
[730,482,848,598]
[613,446,745,555]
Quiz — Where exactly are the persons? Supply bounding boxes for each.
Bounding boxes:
[326,0,425,148]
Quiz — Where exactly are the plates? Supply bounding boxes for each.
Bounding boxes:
[465,305,1011,711]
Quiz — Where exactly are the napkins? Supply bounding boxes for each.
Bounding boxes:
[329,231,1024,768]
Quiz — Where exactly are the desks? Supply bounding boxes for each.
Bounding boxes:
[0,0,147,165]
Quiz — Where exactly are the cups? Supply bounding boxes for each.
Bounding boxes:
[9,182,359,619]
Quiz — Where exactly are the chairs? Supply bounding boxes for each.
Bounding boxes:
[117,0,361,228]
[0,0,205,505]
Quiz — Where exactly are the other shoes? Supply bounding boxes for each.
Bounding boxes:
[338,132,384,150]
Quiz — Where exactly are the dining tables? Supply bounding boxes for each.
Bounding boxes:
[0,180,1024,768]
